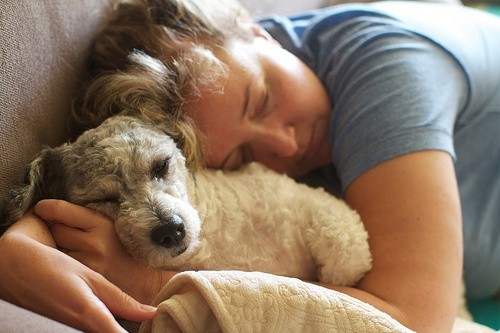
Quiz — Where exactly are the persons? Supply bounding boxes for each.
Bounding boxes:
[0,0,500,332]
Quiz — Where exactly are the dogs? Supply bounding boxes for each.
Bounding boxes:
[0,114,375,287]
